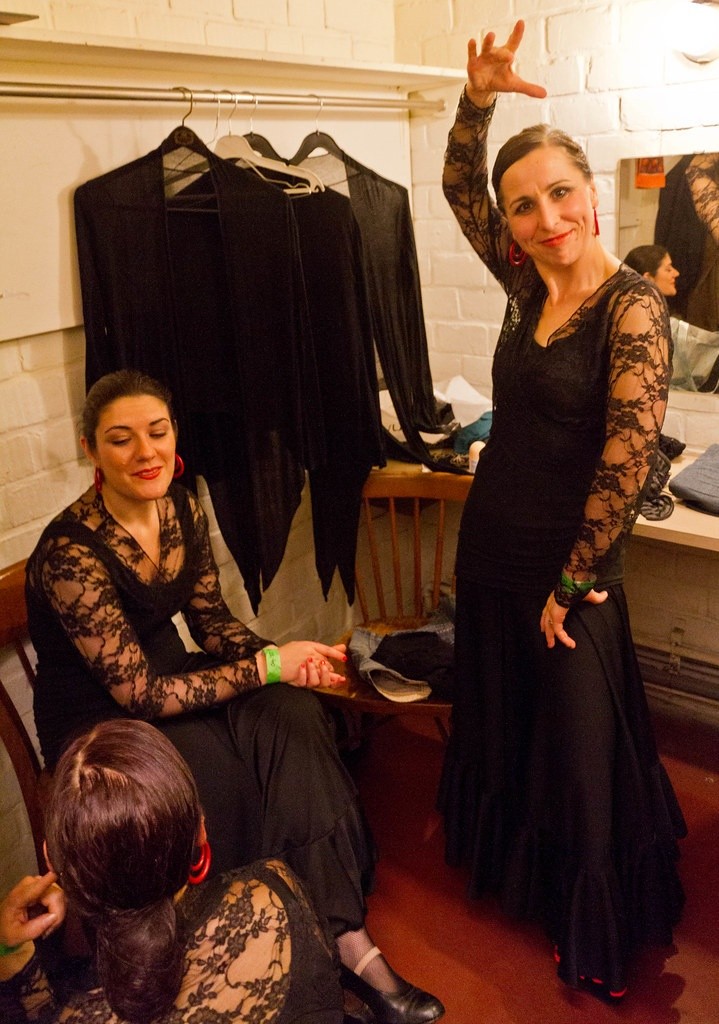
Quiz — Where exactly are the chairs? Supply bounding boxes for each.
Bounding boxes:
[0,555,68,881]
[313,471,473,750]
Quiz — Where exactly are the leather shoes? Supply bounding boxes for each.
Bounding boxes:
[338,945,445,1024]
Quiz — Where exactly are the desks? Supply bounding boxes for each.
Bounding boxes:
[370,452,719,552]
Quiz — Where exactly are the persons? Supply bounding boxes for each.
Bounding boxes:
[25,373,444,1023]
[625,154,716,391]
[0,717,352,1024]
[441,18,688,1005]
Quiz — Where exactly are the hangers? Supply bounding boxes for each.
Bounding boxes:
[78,85,407,213]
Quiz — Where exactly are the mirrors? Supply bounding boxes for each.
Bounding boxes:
[614,152,719,395]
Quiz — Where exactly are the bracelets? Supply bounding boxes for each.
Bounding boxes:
[1,945,19,955]
[561,570,597,594]
[263,645,280,684]
